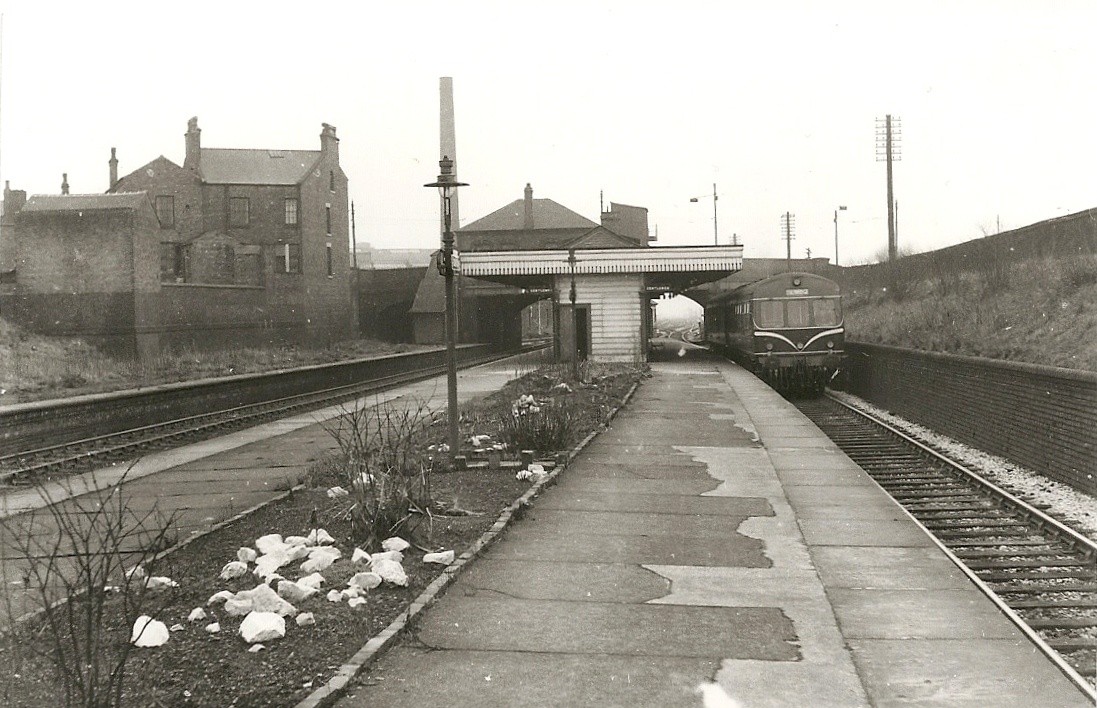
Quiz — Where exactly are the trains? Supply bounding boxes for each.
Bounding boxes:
[704,271,847,395]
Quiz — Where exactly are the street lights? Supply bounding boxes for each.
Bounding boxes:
[424,154,471,457]
[874,114,903,262]
[833,205,847,269]
[778,212,797,257]
[690,183,718,245]
